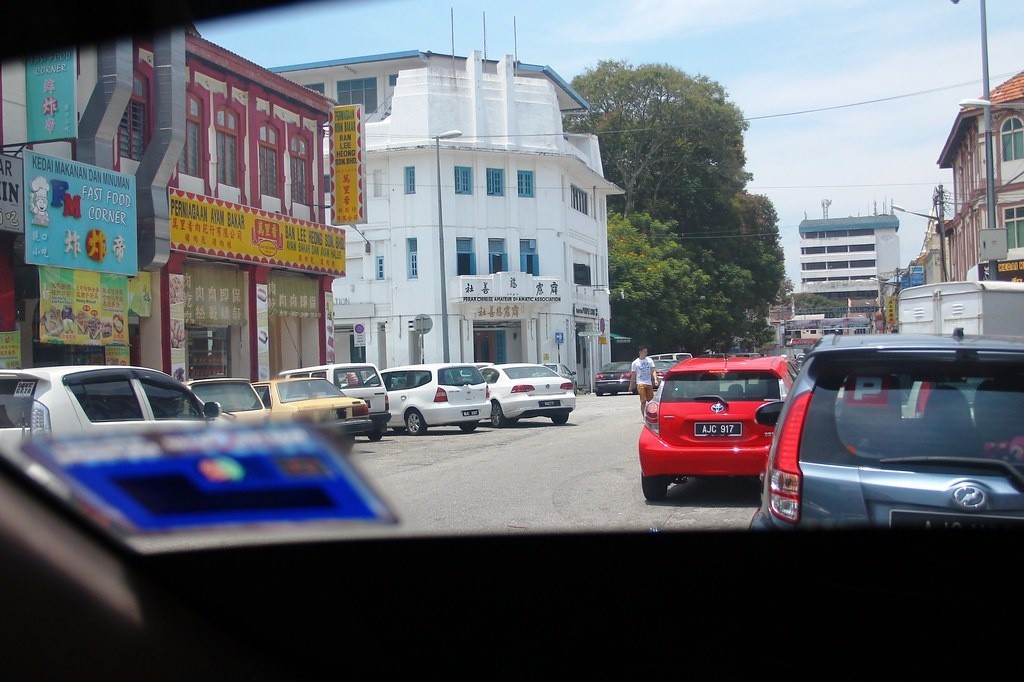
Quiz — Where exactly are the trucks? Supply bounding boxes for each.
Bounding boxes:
[897,281,1024,336]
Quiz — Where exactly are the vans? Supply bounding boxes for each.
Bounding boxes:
[273,362,392,441]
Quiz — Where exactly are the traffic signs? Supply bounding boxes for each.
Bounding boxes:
[555,333,564,344]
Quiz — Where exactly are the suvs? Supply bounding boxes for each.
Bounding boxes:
[0,364,234,450]
[749,327,1024,527]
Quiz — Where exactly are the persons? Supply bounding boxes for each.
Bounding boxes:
[629,344,660,424]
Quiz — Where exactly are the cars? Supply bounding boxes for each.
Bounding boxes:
[638,353,801,502]
[463,363,576,427]
[363,363,492,437]
[250,377,374,455]
[532,362,578,396]
[594,361,639,395]
[646,353,806,391]
[181,378,270,429]
[459,362,496,378]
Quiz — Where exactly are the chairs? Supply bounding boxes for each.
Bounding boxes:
[834,376,1024,459]
[691,374,770,399]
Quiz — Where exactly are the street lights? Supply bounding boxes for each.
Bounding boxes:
[890,183,948,283]
[431,130,462,363]
[870,268,899,298]
[865,299,886,334]
